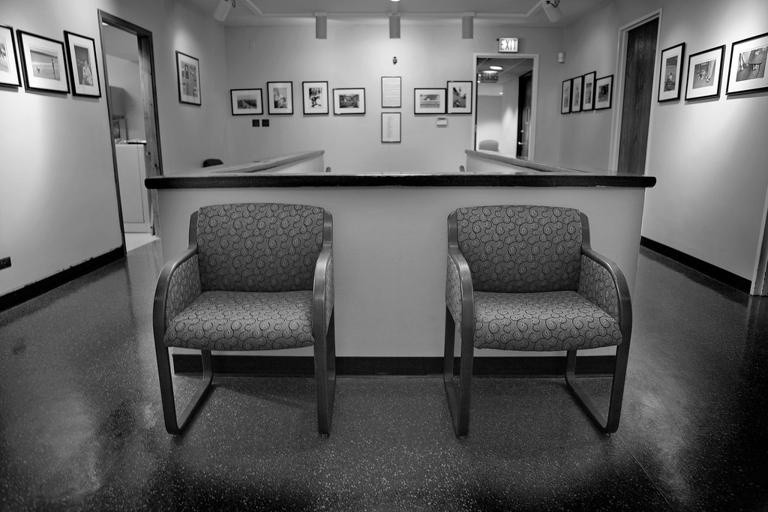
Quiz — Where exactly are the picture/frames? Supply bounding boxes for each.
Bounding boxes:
[560,78,572,113]
[381,76,402,108]
[582,70,594,111]
[18,28,71,94]
[302,81,329,115]
[64,30,105,99]
[594,73,613,110]
[333,87,366,115]
[572,76,582,112]
[656,41,686,102]
[684,44,724,100]
[380,111,401,143]
[177,51,202,106]
[267,81,294,115]
[230,88,263,115]
[414,88,446,114]
[0,24,20,88]
[446,81,472,114]
[725,32,767,93]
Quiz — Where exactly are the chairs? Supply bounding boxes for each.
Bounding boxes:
[445,205,634,441]
[152,201,342,440]
